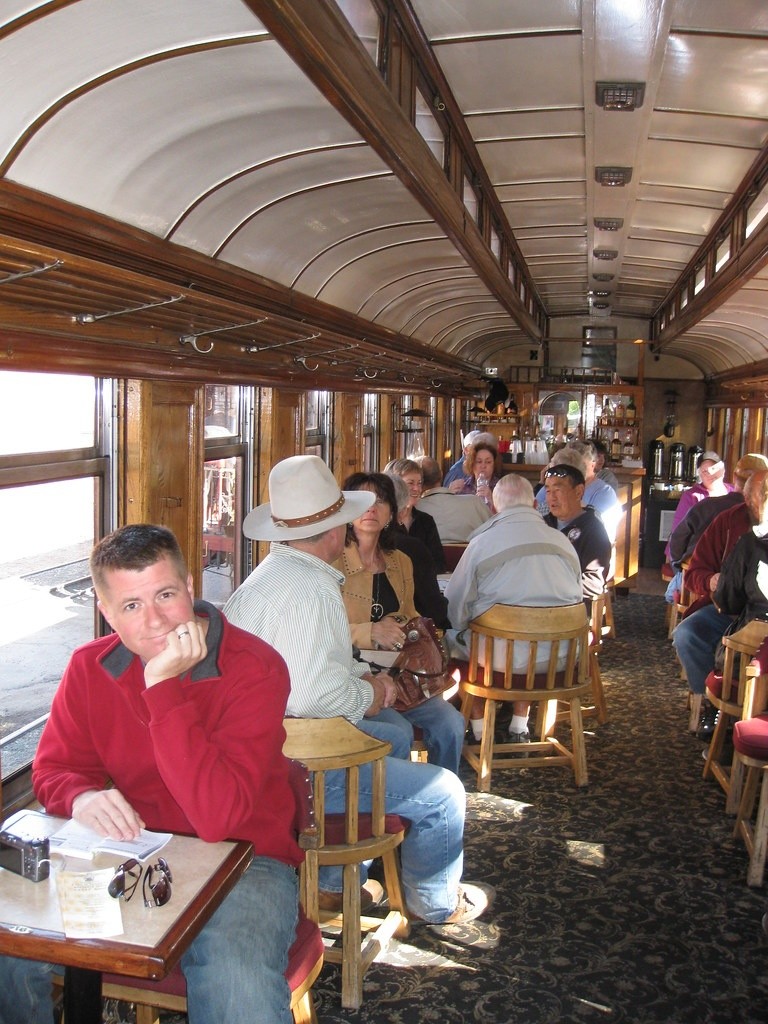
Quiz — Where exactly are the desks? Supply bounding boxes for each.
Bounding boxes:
[0,809,255,1023]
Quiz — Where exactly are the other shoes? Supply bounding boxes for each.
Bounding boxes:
[702,747,732,776]
[697,703,721,741]
[504,730,530,757]
[467,732,497,758]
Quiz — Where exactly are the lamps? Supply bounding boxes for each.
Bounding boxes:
[593,80,645,309]
[664,389,682,437]
[468,407,487,431]
[401,408,432,463]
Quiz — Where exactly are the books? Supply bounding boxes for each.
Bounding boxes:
[48,817,174,862]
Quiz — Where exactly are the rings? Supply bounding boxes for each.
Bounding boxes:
[391,647,396,650]
[393,641,401,648]
[393,690,396,696]
[178,631,189,639]
[400,633,405,637]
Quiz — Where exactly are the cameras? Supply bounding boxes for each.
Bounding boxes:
[0,830,50,882]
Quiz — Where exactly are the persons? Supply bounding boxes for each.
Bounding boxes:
[506,405,518,414]
[383,458,449,573]
[670,471,768,743]
[444,430,482,489]
[448,440,513,516]
[535,449,604,527]
[543,464,610,625]
[665,453,768,604]
[219,454,496,927]
[533,437,617,499]
[536,440,618,583]
[330,471,464,775]
[0,522,306,1024]
[381,472,453,629]
[444,473,582,759]
[683,469,768,622]
[664,450,736,574]
[413,456,494,543]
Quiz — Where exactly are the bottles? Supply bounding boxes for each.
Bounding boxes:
[498,435,507,453]
[604,392,637,426]
[406,420,425,462]
[574,424,583,442]
[497,393,515,422]
[477,472,489,506]
[590,429,596,440]
[601,430,636,462]
[558,427,567,450]
[534,422,541,452]
[510,430,521,451]
[522,425,532,451]
[547,430,556,457]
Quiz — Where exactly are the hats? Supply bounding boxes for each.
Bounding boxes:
[735,453,768,478]
[463,429,483,447]
[243,455,376,542]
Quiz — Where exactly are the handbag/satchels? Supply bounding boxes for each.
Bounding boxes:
[376,616,455,712]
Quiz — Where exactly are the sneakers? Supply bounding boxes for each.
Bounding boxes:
[314,877,383,914]
[405,883,495,924]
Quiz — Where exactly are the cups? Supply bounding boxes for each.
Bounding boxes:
[512,441,549,465]
[673,485,683,491]
[655,483,664,491]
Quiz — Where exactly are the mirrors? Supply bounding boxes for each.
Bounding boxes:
[538,388,584,463]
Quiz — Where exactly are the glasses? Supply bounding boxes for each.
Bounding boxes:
[544,470,577,479]
[697,451,723,468]
[698,464,720,476]
[143,857,172,908]
[403,479,422,488]
[344,522,354,529]
[109,858,143,902]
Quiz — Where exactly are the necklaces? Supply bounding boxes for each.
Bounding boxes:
[365,554,385,619]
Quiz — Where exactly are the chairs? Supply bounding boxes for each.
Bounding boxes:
[51,717,413,1023]
[446,601,591,793]
[673,555,768,887]
